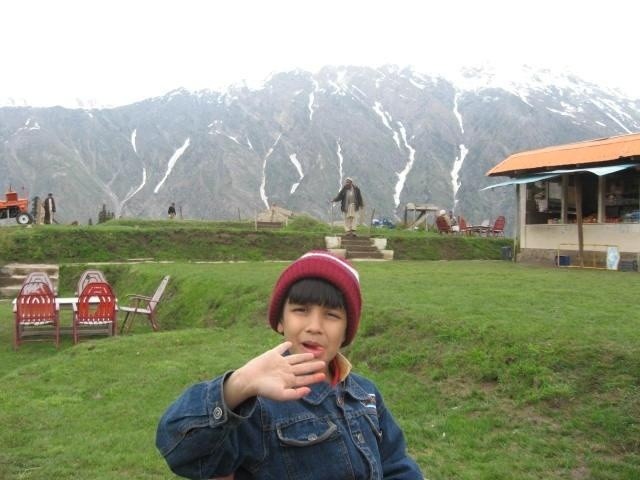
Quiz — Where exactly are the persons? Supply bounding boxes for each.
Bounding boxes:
[604,192,622,219]
[155,249,423,479]
[331,177,365,236]
[448,211,457,226]
[43,192,56,224]
[167,202,177,219]
[440,210,451,233]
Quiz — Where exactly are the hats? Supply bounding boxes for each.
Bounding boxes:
[268,249,362,348]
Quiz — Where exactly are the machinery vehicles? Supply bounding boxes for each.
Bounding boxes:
[0,183,32,225]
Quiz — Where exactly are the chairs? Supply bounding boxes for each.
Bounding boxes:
[15,269,170,348]
[435,215,507,239]
